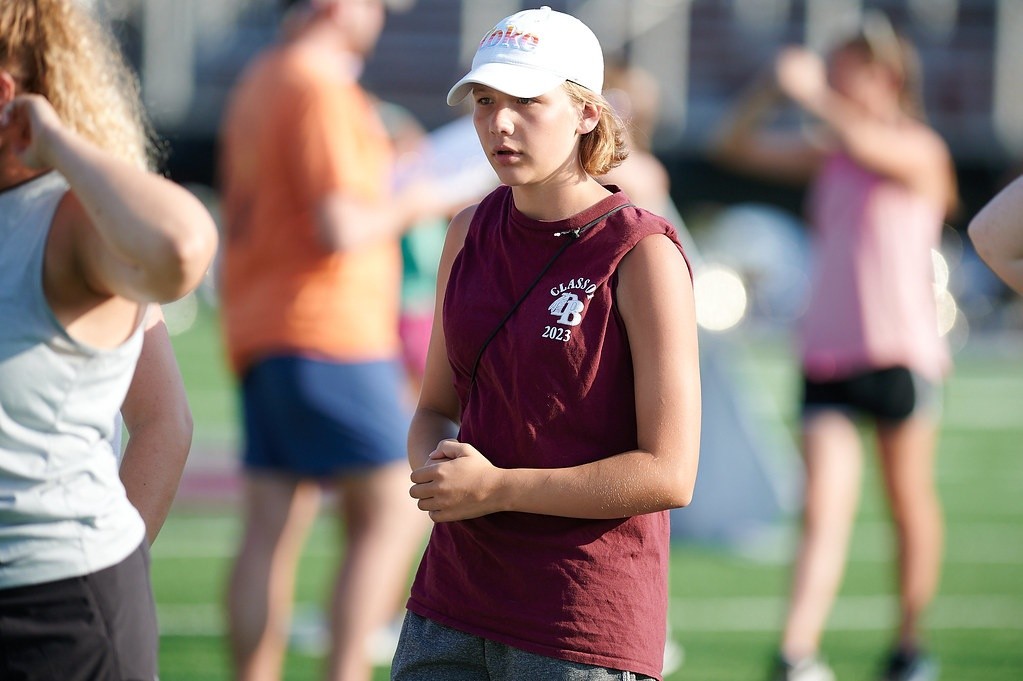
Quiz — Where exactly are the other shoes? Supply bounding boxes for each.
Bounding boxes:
[765,655,837,681]
[883,648,932,681]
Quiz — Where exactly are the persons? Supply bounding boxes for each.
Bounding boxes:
[388,5,702,681]
[0,0,219,681]
[967,176,1023,296]
[706,31,958,681]
[218,0,478,681]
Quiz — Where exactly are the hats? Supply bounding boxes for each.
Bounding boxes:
[445,6,604,107]
